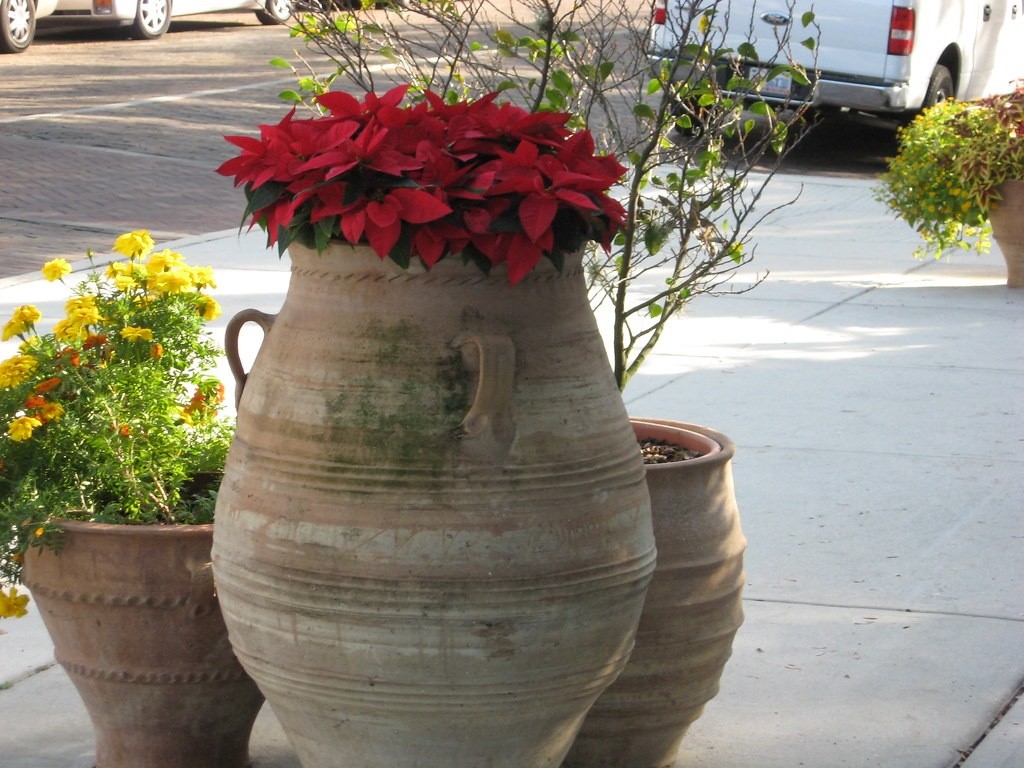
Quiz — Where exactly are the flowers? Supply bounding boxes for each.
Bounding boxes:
[211,85,628,290]
[1,227,237,618]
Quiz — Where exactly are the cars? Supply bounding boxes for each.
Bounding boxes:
[0,0,297,53]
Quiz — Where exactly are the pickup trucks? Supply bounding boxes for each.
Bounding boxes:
[648,0,1024,139]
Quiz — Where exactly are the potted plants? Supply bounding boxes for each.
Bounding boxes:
[870,77,1024,289]
[271,0,824,768]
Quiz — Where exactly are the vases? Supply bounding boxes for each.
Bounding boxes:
[17,516,267,768]
[211,249,658,768]
